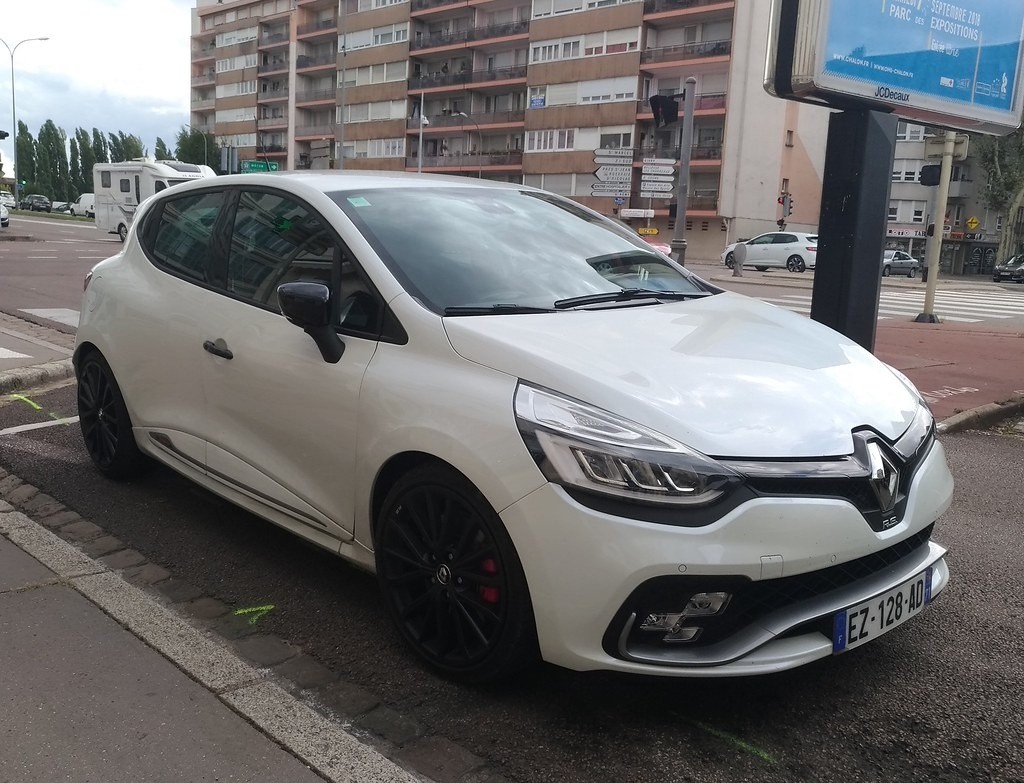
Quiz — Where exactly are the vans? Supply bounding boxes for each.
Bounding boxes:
[69,192,96,217]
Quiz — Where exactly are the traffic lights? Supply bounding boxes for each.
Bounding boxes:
[927,225,934,235]
[778,196,784,204]
[645,92,679,160]
[786,196,793,217]
[917,165,940,187]
[1,131,9,140]
[777,218,784,226]
[21,179,26,185]
[299,153,310,163]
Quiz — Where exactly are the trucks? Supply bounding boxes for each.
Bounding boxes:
[91,159,222,245]
[52,201,75,212]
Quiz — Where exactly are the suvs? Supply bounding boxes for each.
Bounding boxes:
[74,170,963,691]
[20,194,51,213]
[0,191,16,209]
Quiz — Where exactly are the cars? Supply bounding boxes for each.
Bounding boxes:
[64,209,70,214]
[993,253,1024,284]
[719,232,818,274]
[0,203,10,227]
[882,250,919,279]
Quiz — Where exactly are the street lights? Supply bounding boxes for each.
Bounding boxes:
[460,111,485,180]
[185,123,209,166]
[0,34,51,210]
